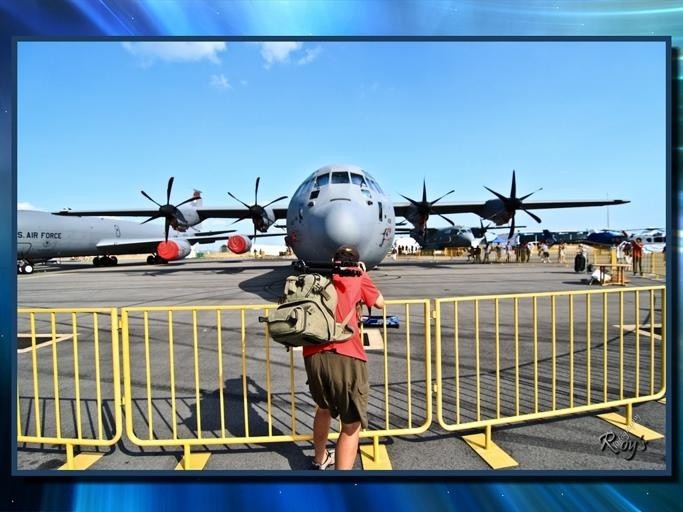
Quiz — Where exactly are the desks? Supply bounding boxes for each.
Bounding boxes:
[592,262,632,286]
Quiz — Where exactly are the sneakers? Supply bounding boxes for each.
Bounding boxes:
[309,450,335,470]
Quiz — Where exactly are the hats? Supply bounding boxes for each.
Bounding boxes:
[333,243,359,263]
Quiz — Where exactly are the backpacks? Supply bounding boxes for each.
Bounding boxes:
[265,268,360,348]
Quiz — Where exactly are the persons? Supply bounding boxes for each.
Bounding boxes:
[304,244,388,471]
[615,237,645,276]
[464,237,567,265]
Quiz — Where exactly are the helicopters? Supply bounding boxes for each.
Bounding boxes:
[628,229,666,252]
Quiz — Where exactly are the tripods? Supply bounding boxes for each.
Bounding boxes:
[302,261,361,277]
